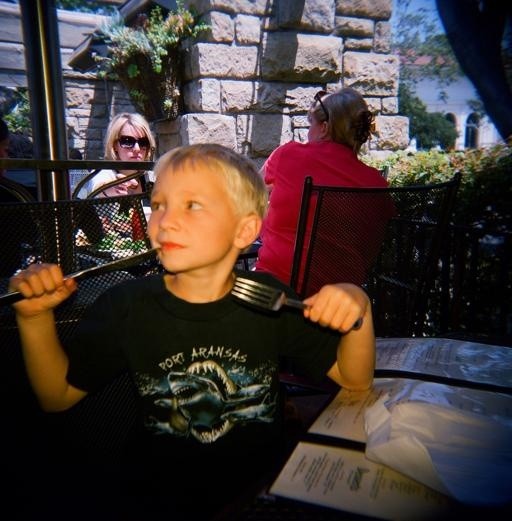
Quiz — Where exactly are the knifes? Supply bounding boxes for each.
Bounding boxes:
[0,248,160,306]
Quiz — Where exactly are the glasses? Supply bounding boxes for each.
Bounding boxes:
[314,90,330,122]
[117,135,150,150]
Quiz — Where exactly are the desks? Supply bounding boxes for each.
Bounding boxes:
[237,330,512,521]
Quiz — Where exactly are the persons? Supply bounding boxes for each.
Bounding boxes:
[86,112,157,239]
[2,118,40,192]
[7,142,378,520]
[255,88,398,301]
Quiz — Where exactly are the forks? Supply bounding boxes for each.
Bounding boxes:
[229,276,363,332]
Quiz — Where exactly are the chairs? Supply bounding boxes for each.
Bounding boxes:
[3,168,481,428]
